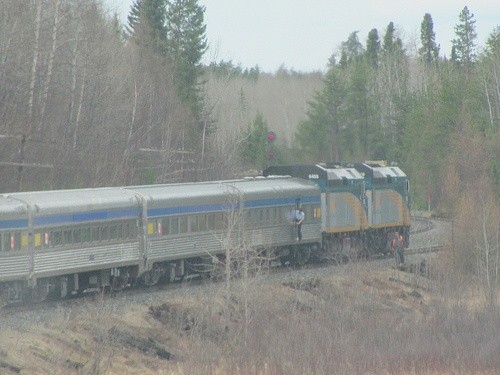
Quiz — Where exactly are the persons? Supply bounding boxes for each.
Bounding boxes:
[296,207,304,240]
[393,231,404,265]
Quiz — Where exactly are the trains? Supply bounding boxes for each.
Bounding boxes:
[0,160,411,308]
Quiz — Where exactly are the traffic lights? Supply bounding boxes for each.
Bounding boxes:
[265,131,276,144]
[266,148,275,158]
